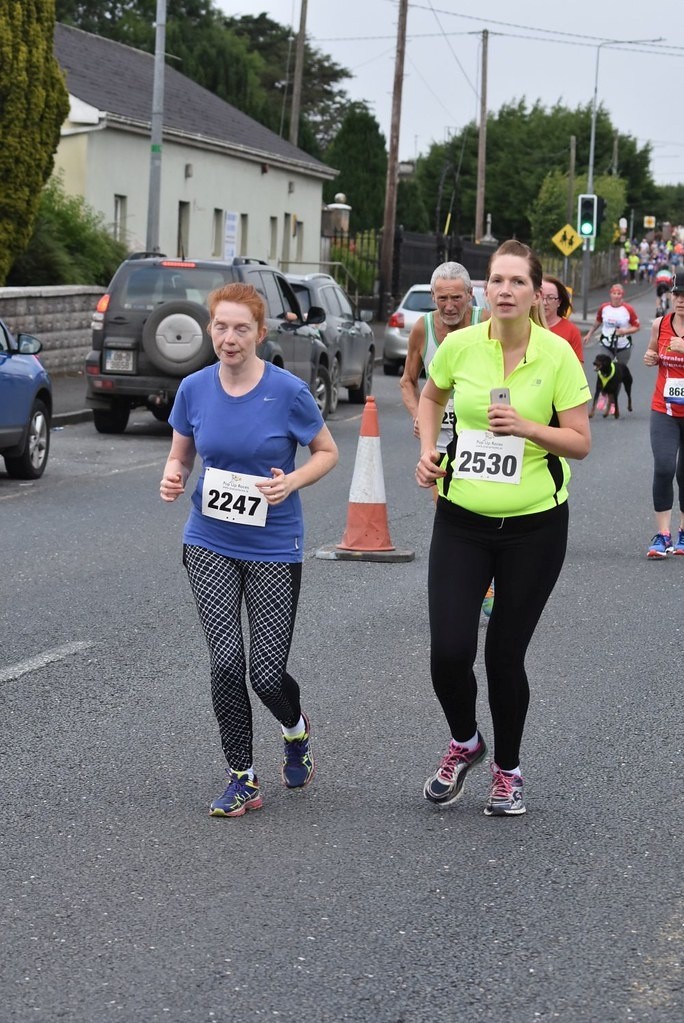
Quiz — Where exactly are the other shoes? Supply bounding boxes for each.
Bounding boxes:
[609,403,616,415]
[597,396,608,410]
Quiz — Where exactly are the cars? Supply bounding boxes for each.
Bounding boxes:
[382,284,488,377]
[0,318,53,481]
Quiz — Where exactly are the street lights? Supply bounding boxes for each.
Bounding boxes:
[583,37,666,320]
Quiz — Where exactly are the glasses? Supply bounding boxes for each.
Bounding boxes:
[542,296,560,304]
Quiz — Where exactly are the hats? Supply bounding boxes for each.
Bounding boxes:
[671,272,684,291]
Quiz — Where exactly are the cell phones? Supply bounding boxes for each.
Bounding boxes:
[488,387,511,437]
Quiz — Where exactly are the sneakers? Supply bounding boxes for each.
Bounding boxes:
[210,768,262,816]
[482,581,494,616]
[647,532,674,557]
[423,729,488,805]
[484,762,527,816]
[673,527,684,554]
[281,710,316,791]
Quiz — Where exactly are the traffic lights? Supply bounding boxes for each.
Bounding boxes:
[577,194,596,238]
[597,196,607,237]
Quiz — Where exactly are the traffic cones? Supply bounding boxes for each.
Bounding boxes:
[316,396,416,564]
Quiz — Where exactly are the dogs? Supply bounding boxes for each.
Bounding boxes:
[588,353,633,419]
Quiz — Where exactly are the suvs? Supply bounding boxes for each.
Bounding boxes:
[84,251,333,435]
[284,274,376,413]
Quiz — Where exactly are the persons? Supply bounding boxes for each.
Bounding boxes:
[159,283,337,818]
[538,276,585,370]
[399,262,492,505]
[414,239,592,816]
[584,284,641,418]
[643,273,684,559]
[617,234,684,318]
